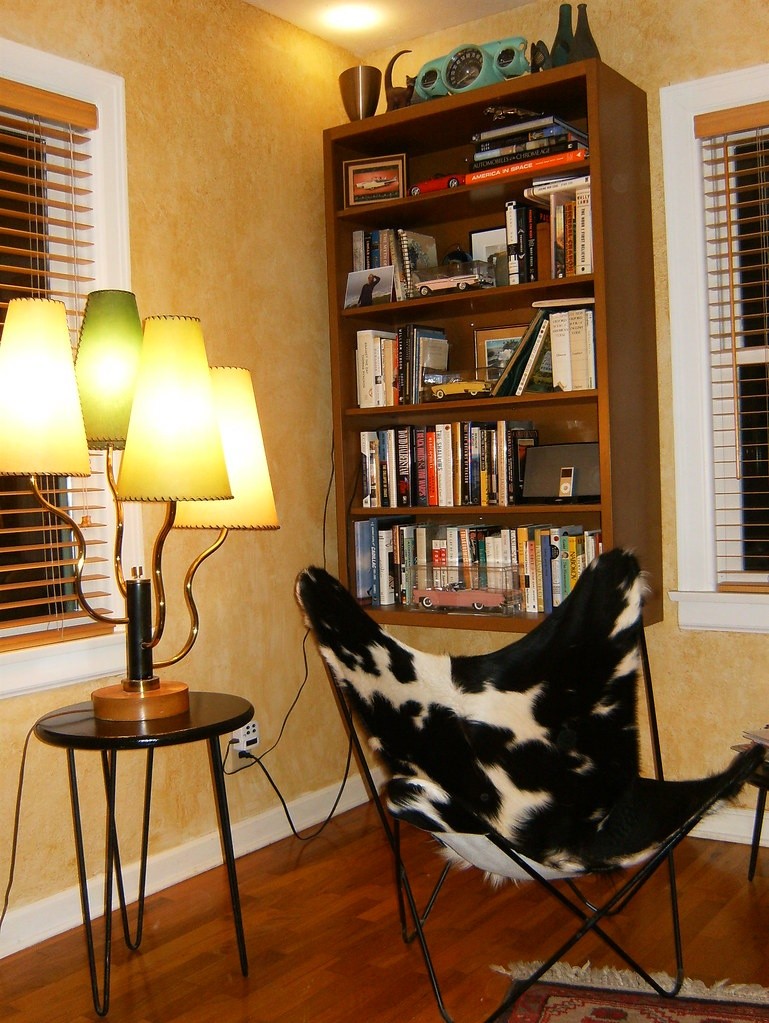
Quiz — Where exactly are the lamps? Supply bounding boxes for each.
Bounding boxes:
[0,290,281,722]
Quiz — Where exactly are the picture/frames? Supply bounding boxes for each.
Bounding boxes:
[468,225,509,286]
[474,324,552,394]
[342,152,407,210]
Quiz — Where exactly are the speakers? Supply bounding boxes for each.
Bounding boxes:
[521,444,603,503]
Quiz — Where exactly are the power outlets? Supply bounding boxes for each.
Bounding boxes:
[231,746,252,772]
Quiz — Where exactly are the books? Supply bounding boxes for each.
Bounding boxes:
[353,228,439,301]
[349,515,501,606]
[360,420,539,509]
[356,323,449,409]
[505,175,594,286]
[489,298,596,397]
[466,114,588,186]
[501,522,602,613]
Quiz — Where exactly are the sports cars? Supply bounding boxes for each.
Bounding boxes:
[412,582,523,612]
[430,376,494,401]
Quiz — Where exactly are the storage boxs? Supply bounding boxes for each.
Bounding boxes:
[406,562,526,617]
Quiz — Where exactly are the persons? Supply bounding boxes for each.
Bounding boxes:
[357,274,380,307]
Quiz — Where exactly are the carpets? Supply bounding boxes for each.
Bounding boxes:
[488,962,769,1022]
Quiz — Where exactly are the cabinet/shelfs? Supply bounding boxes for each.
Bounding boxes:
[323,58,664,634]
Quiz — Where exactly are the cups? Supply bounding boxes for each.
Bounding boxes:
[338,65,381,121]
[496,252,510,287]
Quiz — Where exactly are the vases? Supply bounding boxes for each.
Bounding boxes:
[549,4,600,66]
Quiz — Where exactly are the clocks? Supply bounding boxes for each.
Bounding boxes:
[414,36,531,100]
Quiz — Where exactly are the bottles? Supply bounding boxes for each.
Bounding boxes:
[550,3,573,68]
[572,3,601,65]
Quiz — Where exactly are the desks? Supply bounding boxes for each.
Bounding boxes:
[33,691,254,1017]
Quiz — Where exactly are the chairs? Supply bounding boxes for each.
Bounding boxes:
[292,545,768,1023]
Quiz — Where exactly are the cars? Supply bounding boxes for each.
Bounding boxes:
[407,172,467,197]
[413,272,487,297]
[357,176,398,191]
